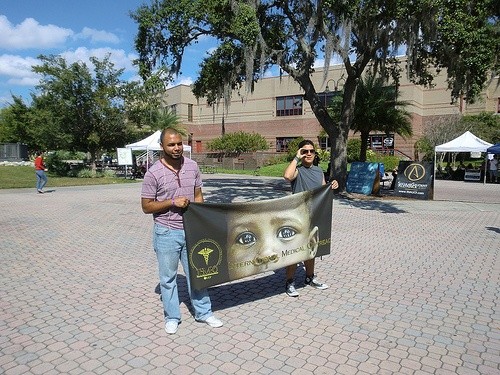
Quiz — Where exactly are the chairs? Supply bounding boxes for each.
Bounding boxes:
[379,172,389,187]
[437,167,465,181]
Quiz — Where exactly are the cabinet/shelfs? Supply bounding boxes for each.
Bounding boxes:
[464,169,481,181]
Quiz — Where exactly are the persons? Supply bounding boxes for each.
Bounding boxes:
[226,191,320,282]
[445,162,451,173]
[487,154,500,183]
[35,151,48,193]
[141,127,223,333]
[458,161,465,173]
[283,140,339,297]
[104,155,111,164]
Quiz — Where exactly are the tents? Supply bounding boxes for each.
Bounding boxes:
[434,131,494,181]
[484,142,500,184]
[126,130,191,176]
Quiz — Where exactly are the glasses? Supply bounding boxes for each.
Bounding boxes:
[303,149,315,154]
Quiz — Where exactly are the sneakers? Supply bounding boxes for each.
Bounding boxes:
[304,276,327,289]
[165,321,177,333]
[201,316,223,327]
[286,279,299,296]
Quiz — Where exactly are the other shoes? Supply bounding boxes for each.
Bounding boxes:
[38,189,44,193]
[495,180,497,182]
[490,180,492,182]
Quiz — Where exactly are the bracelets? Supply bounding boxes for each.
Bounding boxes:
[172,198,174,205]
[294,156,299,162]
